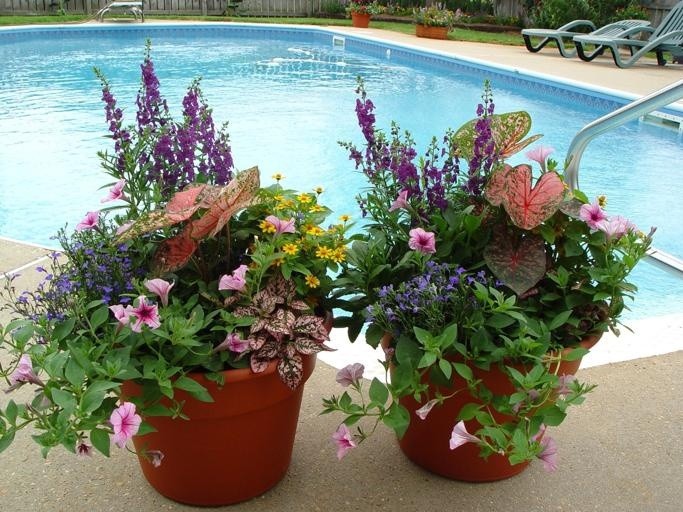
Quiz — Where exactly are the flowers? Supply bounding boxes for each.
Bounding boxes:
[319,71,659,472]
[351,0,377,15]
[415,6,454,26]
[1,33,364,461]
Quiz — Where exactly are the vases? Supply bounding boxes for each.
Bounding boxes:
[115,348,318,504]
[390,330,605,483]
[351,15,369,28]
[416,23,448,39]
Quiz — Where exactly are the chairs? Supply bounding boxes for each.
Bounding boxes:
[521,19,651,58]
[571,0,683,68]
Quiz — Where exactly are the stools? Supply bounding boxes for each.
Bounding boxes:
[97,2,144,22]
